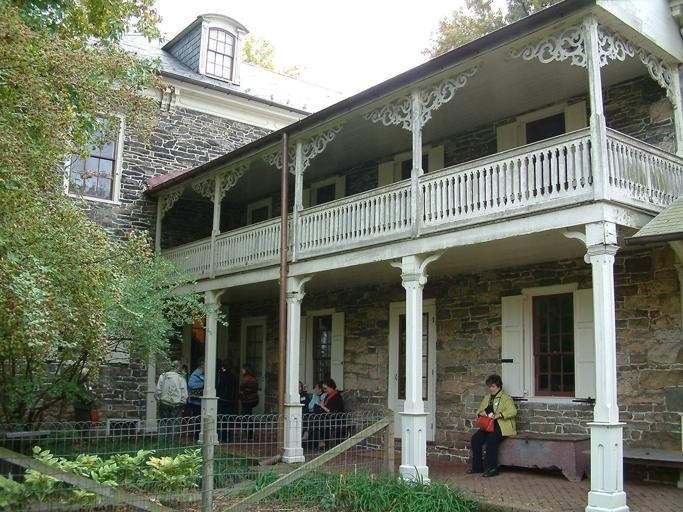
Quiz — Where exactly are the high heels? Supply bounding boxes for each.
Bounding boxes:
[314,446,325,451]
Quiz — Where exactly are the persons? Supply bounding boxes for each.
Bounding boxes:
[153,359,258,444]
[299,381,310,416]
[464,373,518,478]
[300,379,346,450]
[302,382,328,417]
[73,367,96,435]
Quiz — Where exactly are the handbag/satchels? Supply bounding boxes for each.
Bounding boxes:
[242,393,258,407]
[477,417,495,433]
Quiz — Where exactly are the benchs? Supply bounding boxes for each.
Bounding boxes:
[581,447,683,488]
[481,431,590,484]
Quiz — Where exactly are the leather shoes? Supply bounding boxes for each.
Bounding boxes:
[483,468,499,477]
[467,469,483,474]
